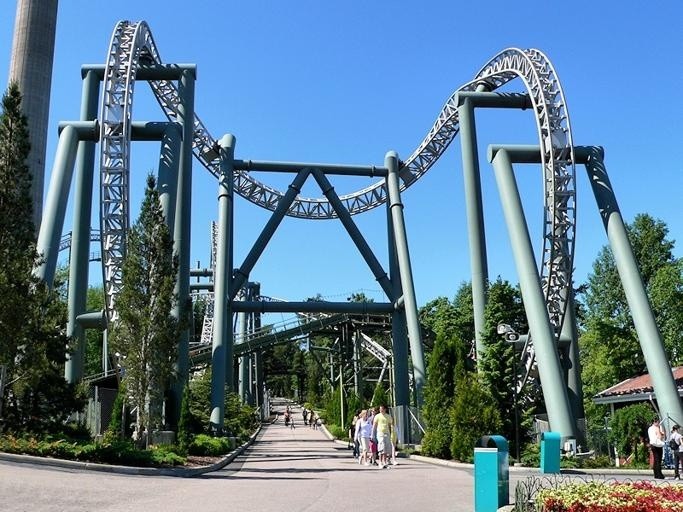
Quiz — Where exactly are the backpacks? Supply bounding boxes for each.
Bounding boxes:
[669,438,681,450]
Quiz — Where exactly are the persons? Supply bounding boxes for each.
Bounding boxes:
[348,404,399,469]
[284,406,321,430]
[648,415,683,480]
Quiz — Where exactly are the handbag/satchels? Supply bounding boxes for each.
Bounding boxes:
[369,441,376,453]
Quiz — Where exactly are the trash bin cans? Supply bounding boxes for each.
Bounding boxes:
[473,435,509,512]
[540,432,561,474]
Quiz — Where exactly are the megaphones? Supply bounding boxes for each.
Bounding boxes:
[505,333,521,342]
[497,324,511,334]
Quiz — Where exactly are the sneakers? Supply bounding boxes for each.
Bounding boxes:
[655,475,665,479]
[354,456,399,468]
